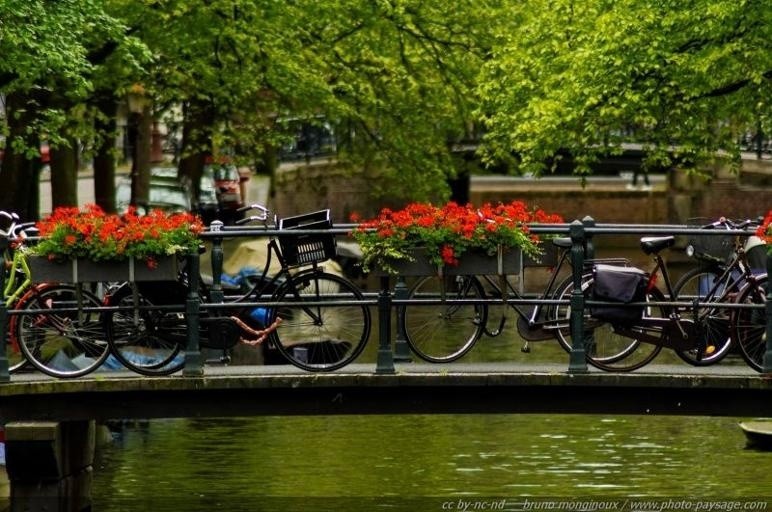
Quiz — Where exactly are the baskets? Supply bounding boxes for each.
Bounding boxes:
[279,208,338,266]
[686,217,738,266]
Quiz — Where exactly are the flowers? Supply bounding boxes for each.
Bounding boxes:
[754,207,772,257]
[33,203,204,270]
[348,200,566,275]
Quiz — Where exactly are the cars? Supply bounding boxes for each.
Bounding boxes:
[116,153,251,222]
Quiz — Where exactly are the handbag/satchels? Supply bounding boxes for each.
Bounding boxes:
[589,264,645,327]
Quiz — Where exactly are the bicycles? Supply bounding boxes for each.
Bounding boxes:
[0,209,770,381]
